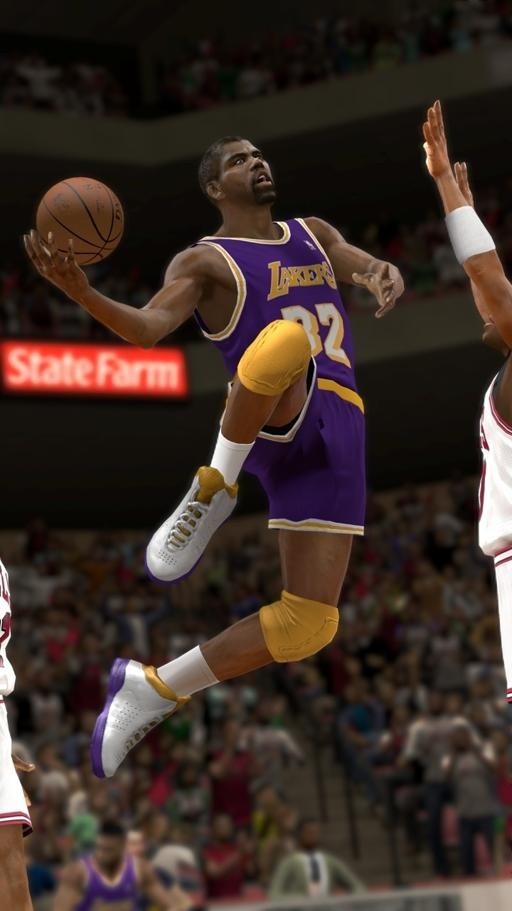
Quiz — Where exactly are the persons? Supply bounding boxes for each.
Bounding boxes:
[1,562,37,909]
[0,473,509,909]
[21,124,407,780]
[420,99,512,709]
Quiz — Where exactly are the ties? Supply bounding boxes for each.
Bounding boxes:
[311,855,323,882]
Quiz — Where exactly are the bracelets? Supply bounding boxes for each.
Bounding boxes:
[442,203,496,267]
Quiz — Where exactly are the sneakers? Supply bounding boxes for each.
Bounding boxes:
[141,462,240,584]
[87,654,194,780]
